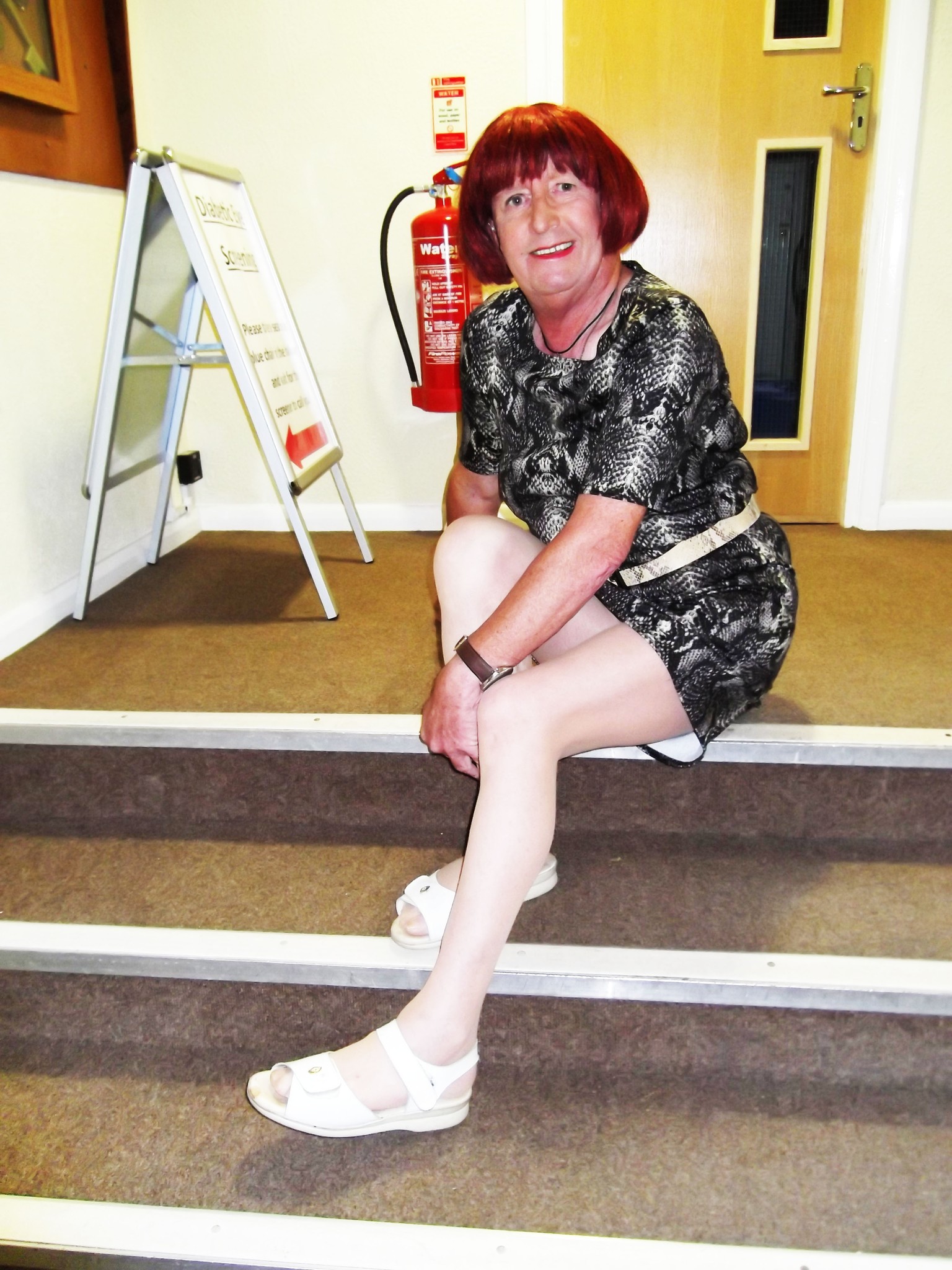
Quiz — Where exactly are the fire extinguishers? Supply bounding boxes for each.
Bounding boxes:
[380,161,486,413]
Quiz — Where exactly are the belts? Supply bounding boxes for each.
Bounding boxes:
[604,494,759,586]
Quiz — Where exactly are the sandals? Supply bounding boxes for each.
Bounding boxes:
[390,850,558,949]
[246,1019,479,1138]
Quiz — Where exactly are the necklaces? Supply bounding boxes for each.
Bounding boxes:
[524,286,618,357]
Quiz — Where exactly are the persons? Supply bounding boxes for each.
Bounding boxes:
[241,99,800,1135]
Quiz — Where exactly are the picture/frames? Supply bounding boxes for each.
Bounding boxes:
[0,0,77,115]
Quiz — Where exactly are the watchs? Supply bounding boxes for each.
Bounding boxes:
[452,634,514,694]
